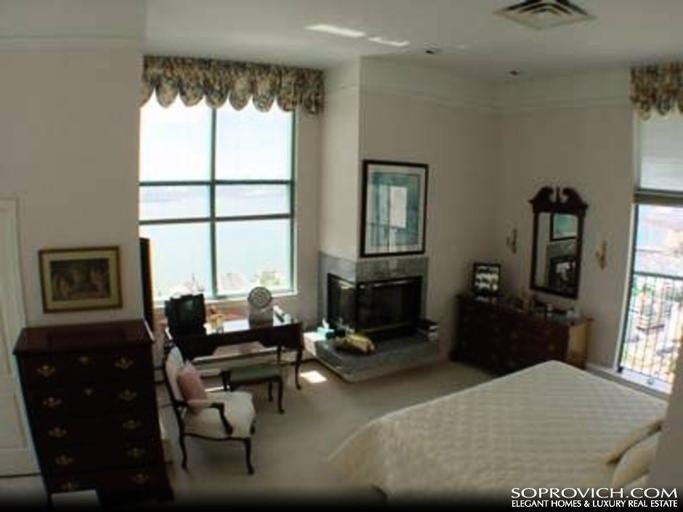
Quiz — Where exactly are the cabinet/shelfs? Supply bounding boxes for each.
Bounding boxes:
[13,318,172,509]
[451,289,594,378]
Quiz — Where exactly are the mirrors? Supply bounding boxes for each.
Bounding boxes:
[527,184,587,299]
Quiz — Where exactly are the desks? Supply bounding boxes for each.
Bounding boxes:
[163,304,302,392]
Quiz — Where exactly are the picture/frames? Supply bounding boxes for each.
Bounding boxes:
[37,245,124,314]
[360,159,428,257]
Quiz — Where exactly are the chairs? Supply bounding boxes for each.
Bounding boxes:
[163,345,256,476]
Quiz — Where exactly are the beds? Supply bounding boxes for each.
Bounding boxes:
[326,341,683,508]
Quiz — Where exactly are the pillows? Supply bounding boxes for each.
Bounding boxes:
[607,421,662,499]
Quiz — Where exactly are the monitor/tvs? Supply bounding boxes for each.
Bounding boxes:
[165,294,206,337]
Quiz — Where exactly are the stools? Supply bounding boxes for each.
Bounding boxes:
[219,361,286,416]
